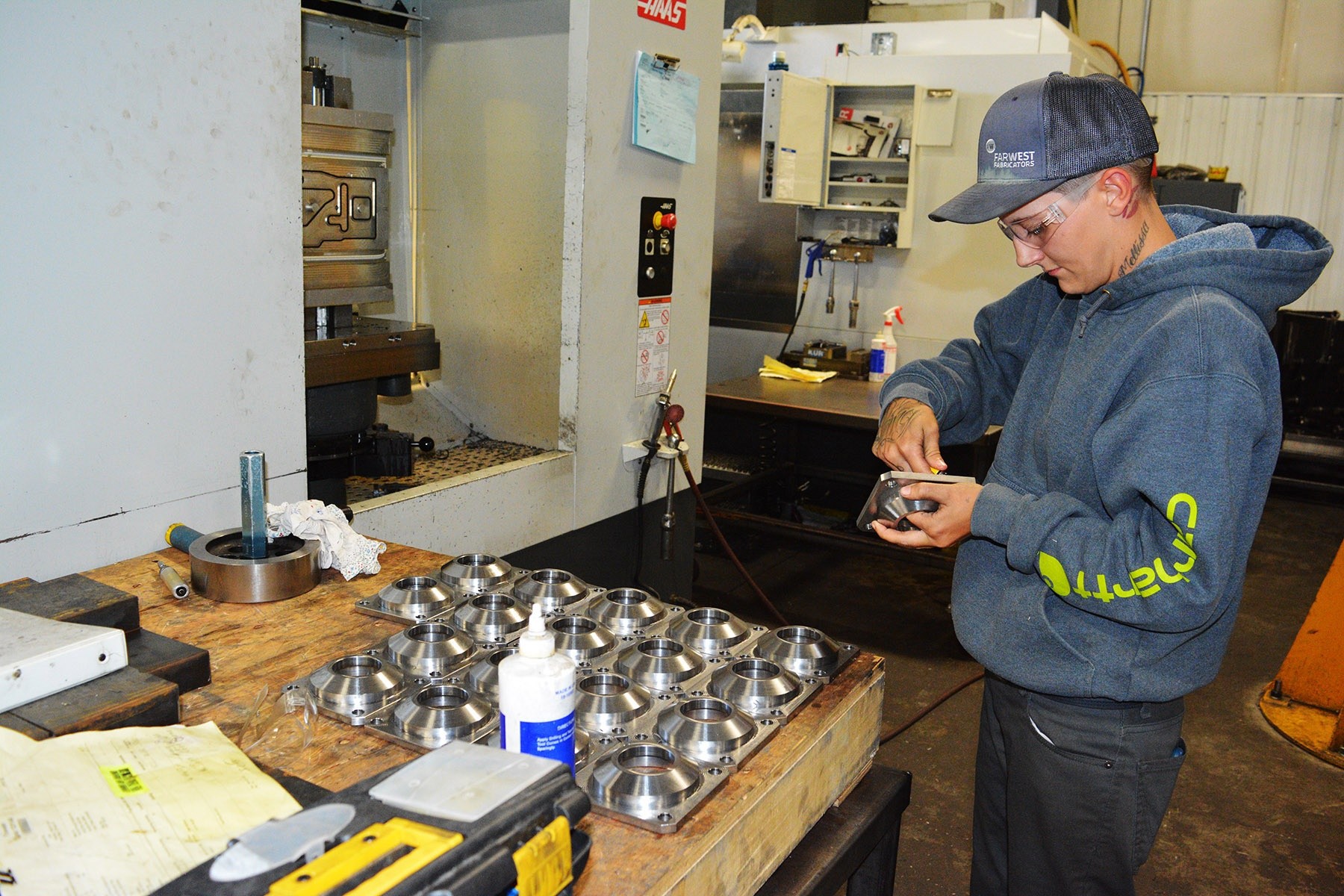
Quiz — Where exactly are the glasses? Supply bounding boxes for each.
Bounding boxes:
[997,155,1152,250]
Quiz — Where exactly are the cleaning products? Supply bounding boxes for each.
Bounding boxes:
[883,305,905,378]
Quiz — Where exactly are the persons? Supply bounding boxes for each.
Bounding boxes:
[870,70,1335,895]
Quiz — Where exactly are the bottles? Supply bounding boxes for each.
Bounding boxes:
[868,330,886,382]
[498,603,577,782]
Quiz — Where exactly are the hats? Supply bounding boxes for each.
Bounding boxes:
[927,71,1160,224]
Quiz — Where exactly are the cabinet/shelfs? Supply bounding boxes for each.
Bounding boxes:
[697,372,1003,570]
[758,70,924,248]
